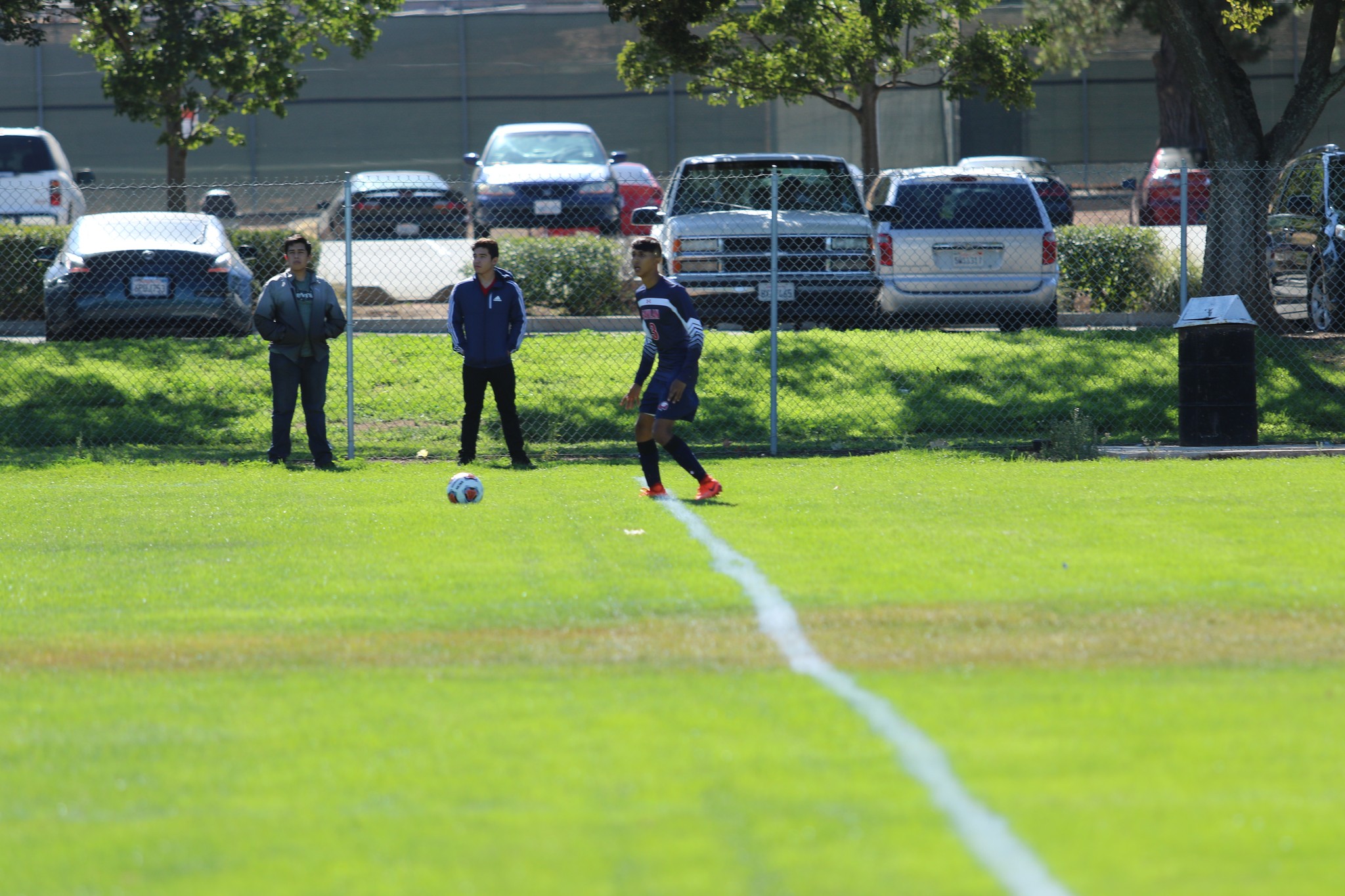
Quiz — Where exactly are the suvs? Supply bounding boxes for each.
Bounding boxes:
[1265,143,1345,334]
[0,125,97,226]
[629,153,905,330]
[865,165,1059,330]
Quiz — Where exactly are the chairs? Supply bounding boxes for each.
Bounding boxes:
[953,191,1004,219]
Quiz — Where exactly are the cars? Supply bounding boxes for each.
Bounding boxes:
[31,210,260,342]
[544,160,664,237]
[1122,145,1212,226]
[463,122,629,240]
[940,156,1073,228]
[316,169,469,241]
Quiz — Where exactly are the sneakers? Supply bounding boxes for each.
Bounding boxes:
[639,482,669,497]
[695,479,723,500]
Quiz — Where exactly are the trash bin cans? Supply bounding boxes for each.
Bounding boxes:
[1123,179,1135,190]
[1173,294,1260,446]
[202,189,236,217]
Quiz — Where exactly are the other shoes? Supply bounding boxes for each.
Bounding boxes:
[315,460,340,471]
[458,449,474,466]
[270,456,286,465]
[512,451,538,467]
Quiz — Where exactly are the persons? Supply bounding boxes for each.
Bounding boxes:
[620,238,723,500]
[254,234,347,470]
[448,238,539,471]
